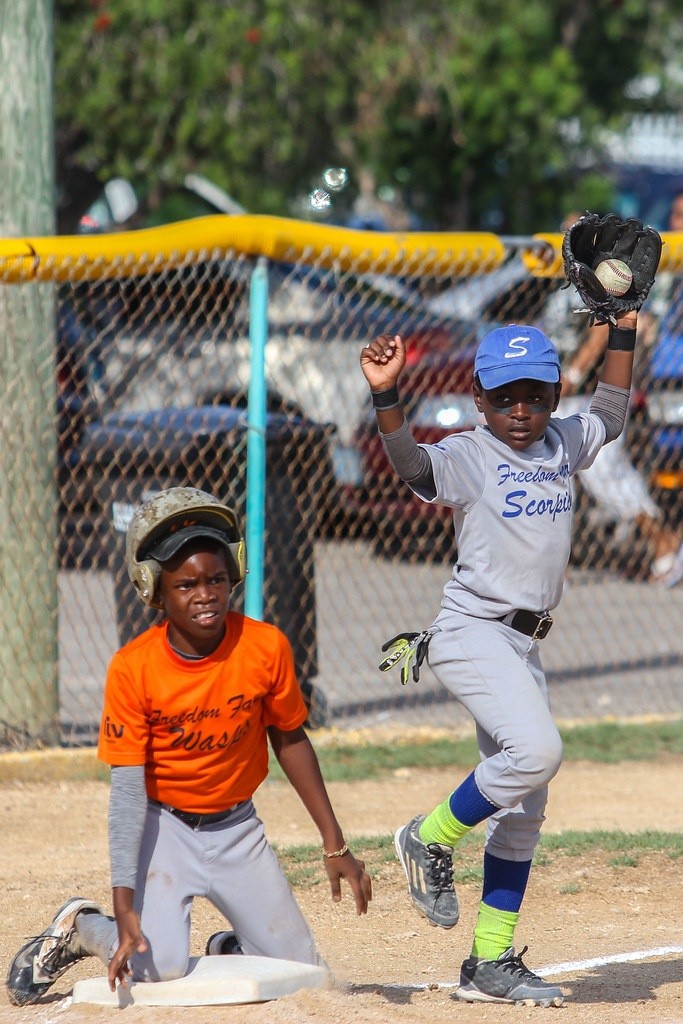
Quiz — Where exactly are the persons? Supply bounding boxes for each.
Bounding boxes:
[360,308,638,1013]
[533,208,682,594]
[8,538,372,1007]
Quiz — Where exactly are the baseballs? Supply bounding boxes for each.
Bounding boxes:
[592,258,634,297]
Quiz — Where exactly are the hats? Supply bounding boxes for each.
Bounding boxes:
[474,324,561,390]
[148,516,232,561]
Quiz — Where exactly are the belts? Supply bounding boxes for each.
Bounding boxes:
[497,610,553,638]
[164,803,241,826]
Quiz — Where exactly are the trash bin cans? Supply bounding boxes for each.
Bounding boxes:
[82,405,329,733]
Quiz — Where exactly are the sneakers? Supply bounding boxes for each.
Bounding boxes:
[452,946,563,1007]
[393,813,459,929]
[207,930,237,955]
[7,897,102,1007]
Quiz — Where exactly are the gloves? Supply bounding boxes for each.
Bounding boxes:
[378,630,433,685]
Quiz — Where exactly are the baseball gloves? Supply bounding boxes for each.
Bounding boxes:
[560,208,666,323]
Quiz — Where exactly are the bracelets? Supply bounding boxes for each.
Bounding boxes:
[320,840,348,858]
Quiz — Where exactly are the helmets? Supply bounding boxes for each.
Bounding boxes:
[125,487,244,608]
